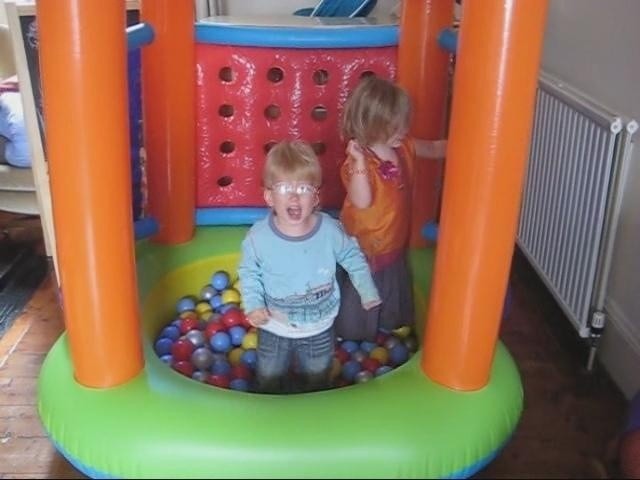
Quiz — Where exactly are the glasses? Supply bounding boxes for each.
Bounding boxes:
[266,181,320,194]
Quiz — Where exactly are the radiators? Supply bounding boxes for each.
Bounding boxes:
[516,70,639,381]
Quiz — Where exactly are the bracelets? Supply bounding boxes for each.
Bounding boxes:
[347,168,371,175]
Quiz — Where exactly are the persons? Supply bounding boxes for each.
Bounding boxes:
[337,77,448,342]
[238,140,382,393]
[0,74,33,168]
